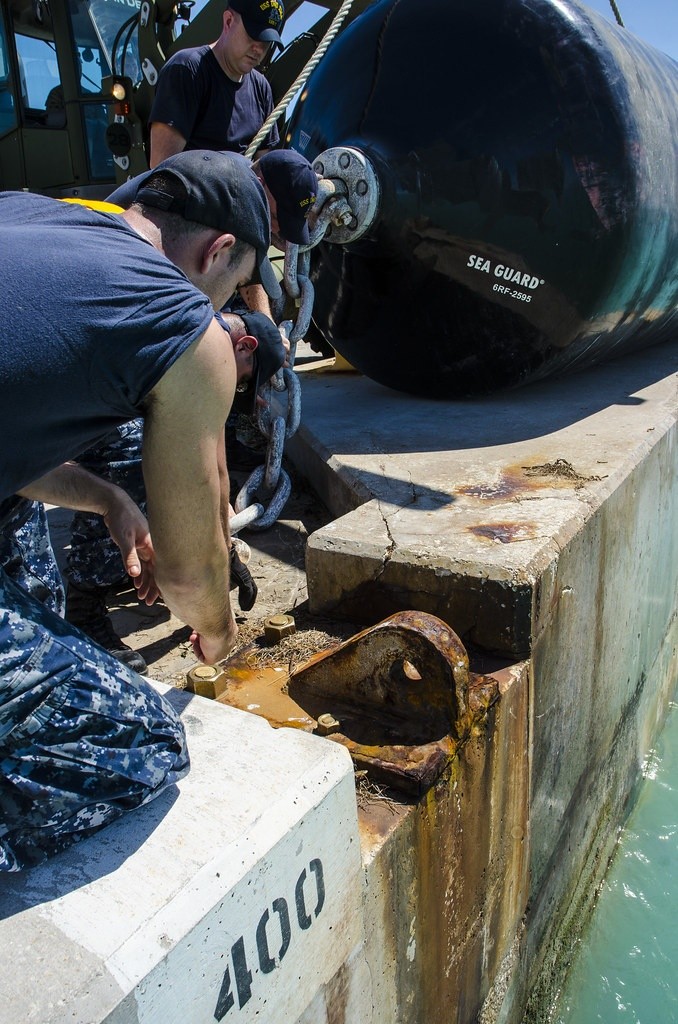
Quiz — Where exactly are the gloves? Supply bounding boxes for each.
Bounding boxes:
[227,544,258,611]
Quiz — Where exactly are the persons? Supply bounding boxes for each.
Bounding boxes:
[148,0,287,169]
[0,152,323,880]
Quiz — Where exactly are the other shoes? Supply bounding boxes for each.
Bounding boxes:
[63,595,149,677]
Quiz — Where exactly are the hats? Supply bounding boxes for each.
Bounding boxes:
[134,149,283,300]
[233,308,286,410]
[231,0,285,53]
[261,149,319,245]
